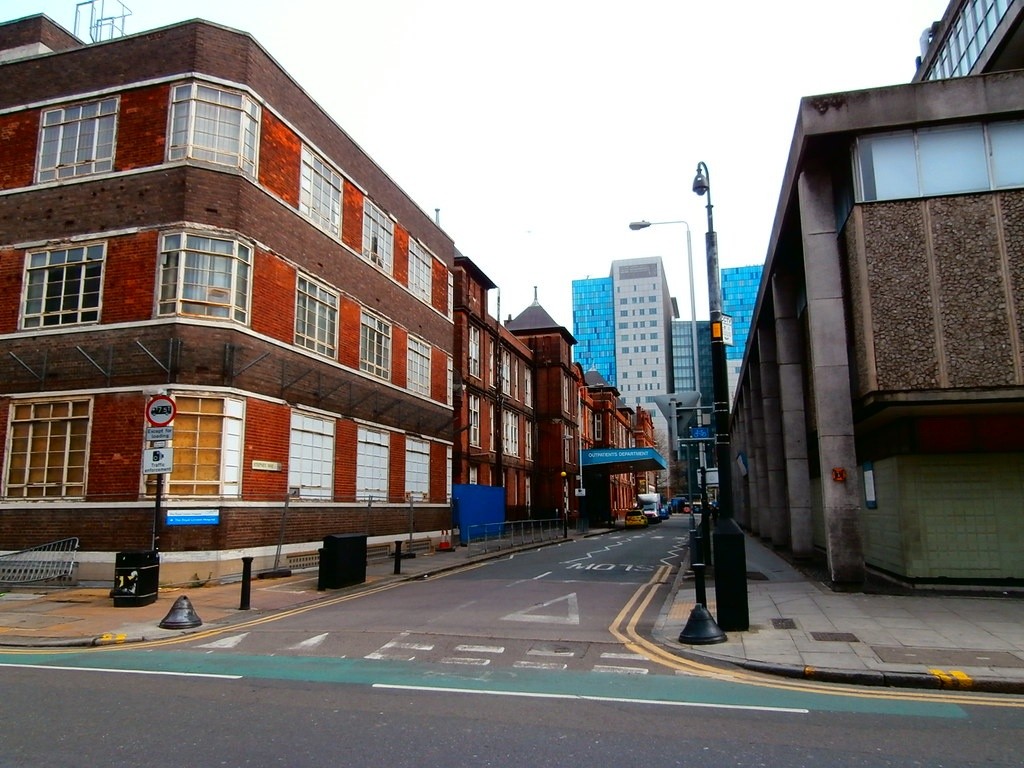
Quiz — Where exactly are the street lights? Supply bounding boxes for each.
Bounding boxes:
[629,220,728,644]
[692,160,750,633]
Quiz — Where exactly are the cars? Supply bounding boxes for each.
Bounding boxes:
[660,503,700,520]
[625,509,648,529]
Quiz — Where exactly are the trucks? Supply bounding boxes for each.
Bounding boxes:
[637,493,662,524]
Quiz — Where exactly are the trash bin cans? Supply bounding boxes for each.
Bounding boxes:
[113,550,161,608]
[322,532,369,589]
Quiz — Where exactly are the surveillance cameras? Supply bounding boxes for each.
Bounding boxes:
[692,174,708,196]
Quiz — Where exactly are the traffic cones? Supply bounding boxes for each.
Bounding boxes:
[437,527,450,551]
